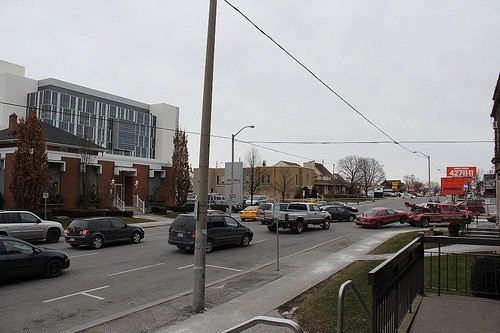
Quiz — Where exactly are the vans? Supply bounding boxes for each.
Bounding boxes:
[167,213,254,255]
[246,194,268,205]
[383,192,397,197]
[208,192,221,203]
[65,216,147,250]
[213,196,228,205]
[0,210,65,244]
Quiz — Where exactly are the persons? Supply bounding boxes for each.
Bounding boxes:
[428,198,440,202]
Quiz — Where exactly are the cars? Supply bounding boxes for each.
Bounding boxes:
[484,211,497,223]
[400,193,417,199]
[353,207,409,229]
[453,199,486,216]
[412,193,423,197]
[239,206,260,222]
[320,205,357,222]
[319,201,360,213]
[0,235,70,285]
[255,202,275,225]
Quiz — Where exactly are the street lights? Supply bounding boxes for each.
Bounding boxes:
[229,124,255,214]
[412,150,433,202]
[373,163,385,192]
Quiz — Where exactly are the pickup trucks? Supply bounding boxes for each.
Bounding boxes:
[405,202,476,228]
[263,201,333,234]
